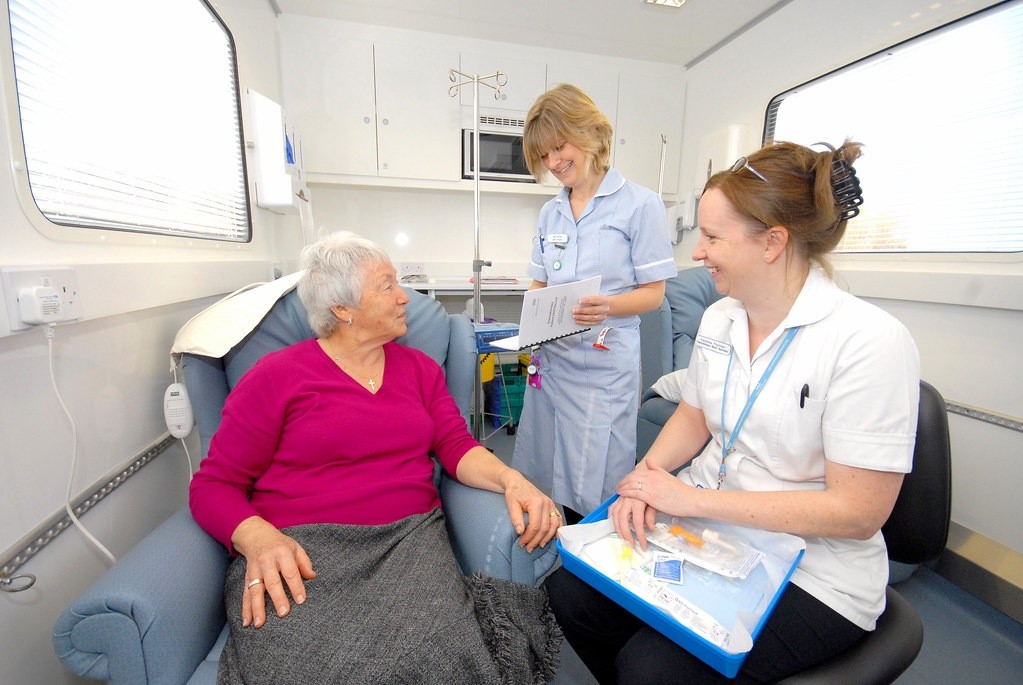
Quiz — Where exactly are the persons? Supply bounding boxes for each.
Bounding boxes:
[188,231,564,685]
[510,82,679,526]
[543,140,921,685]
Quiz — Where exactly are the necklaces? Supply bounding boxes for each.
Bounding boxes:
[329,340,382,392]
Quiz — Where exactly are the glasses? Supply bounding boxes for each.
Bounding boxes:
[730,156,771,182]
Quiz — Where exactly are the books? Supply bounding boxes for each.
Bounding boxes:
[489,274,602,351]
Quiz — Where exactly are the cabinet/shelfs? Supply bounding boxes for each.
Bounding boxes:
[276,14,688,206]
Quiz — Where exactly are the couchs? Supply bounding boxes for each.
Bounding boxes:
[634,261,729,475]
[53,283,565,685]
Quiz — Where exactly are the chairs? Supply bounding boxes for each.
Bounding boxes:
[540,372,956,685]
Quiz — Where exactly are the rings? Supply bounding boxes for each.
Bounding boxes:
[638,481,642,490]
[595,314,598,321]
[247,578,261,588]
[549,511,560,517]
